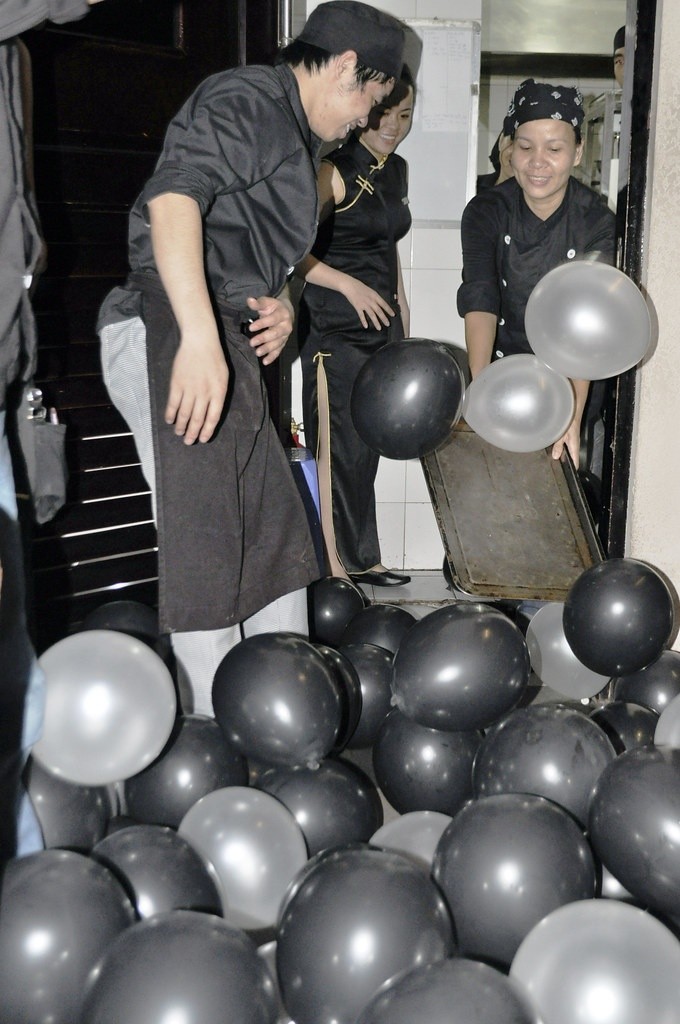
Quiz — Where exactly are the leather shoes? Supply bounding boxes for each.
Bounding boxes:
[349,569,411,588]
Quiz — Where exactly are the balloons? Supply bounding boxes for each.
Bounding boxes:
[524,260,653,380]
[350,338,467,460]
[32,630,177,787]
[0,576,679,1024]
[564,559,674,681]
[527,602,611,701]
[461,353,575,453]
[392,601,530,730]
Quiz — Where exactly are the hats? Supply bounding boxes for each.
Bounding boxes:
[614,26,625,52]
[295,0,407,82]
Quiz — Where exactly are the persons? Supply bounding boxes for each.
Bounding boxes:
[98,0,424,721]
[293,63,417,587]
[475,124,515,196]
[602,193,620,260]
[442,77,604,620]
[0,0,94,868]
[614,24,625,89]
